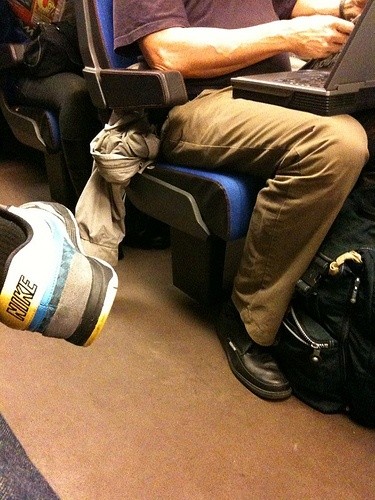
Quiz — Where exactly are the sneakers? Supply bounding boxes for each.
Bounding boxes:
[0,200,118,347]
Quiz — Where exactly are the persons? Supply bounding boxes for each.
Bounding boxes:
[1,0,102,200]
[110,0,368,400]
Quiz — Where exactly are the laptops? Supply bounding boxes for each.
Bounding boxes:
[228,1,375,117]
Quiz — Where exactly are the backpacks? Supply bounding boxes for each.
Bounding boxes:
[269,190,375,430]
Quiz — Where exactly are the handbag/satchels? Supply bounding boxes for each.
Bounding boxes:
[22,21,84,80]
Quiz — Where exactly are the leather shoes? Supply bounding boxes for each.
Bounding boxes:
[123,218,170,249]
[214,302,292,400]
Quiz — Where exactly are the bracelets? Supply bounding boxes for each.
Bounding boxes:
[338,0,345,20]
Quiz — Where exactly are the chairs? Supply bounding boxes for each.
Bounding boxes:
[74,0,265,306]
[1,80,82,215]
